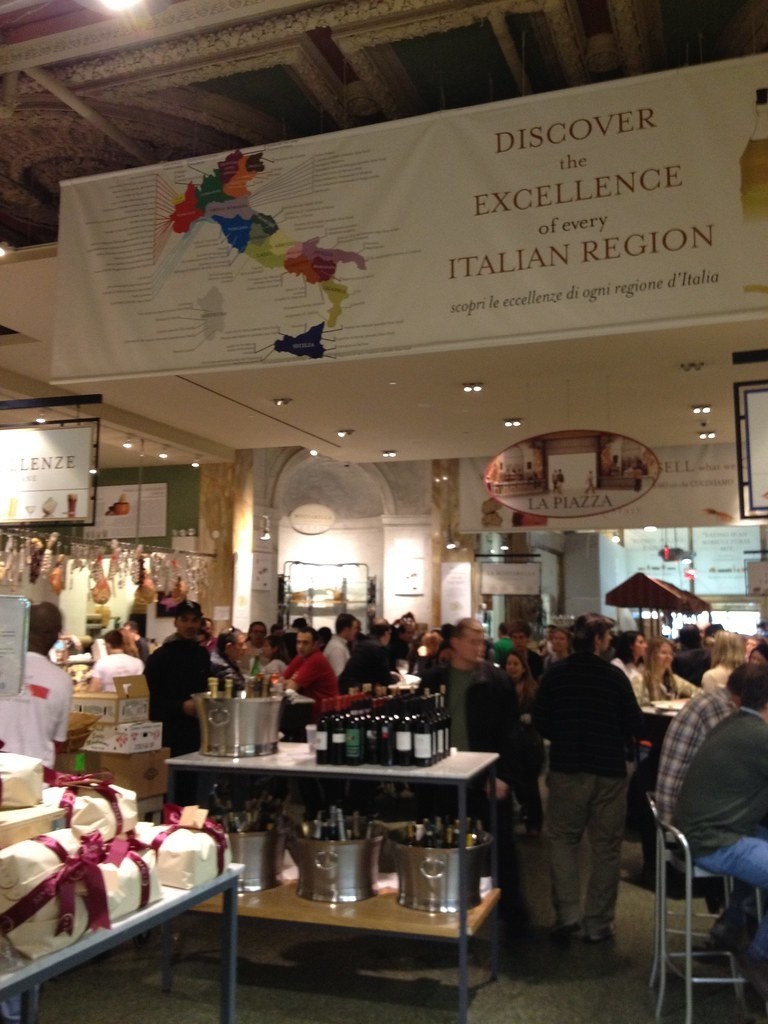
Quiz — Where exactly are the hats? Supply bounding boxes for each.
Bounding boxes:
[174,601,202,617]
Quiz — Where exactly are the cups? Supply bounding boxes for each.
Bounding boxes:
[305,724,316,752]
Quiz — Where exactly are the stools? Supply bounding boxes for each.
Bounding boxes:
[641,793,768,1024]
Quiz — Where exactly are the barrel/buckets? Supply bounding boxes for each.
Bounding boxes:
[285,821,390,904]
[190,691,291,758]
[208,811,305,894]
[385,827,495,914]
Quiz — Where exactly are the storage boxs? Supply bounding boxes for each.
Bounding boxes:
[49,671,168,798]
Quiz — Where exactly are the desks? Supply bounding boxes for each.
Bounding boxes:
[0,861,240,1024]
[165,747,500,1024]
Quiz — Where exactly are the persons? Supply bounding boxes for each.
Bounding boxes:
[85,620,149,697]
[552,468,564,494]
[530,612,642,942]
[140,600,768,1005]
[626,458,648,493]
[584,470,595,493]
[0,600,71,769]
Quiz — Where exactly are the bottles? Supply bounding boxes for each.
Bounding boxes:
[316,683,452,767]
[208,793,290,834]
[311,805,376,842]
[207,653,284,698]
[405,816,484,849]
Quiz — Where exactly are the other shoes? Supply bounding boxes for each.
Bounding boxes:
[547,917,585,940]
[586,922,617,943]
